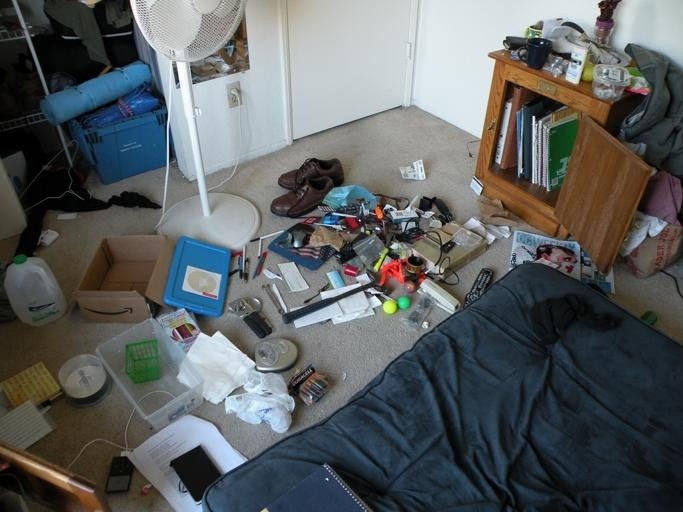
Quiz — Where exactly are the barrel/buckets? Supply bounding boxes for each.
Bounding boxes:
[4,255,67,328]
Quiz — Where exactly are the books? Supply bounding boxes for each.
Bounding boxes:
[508,229,581,282]
[492,84,580,195]
[580,247,616,297]
[259,463,374,512]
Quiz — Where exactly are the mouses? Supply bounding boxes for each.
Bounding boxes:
[291,229,308,249]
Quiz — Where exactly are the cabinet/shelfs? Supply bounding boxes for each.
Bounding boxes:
[0,1,81,190]
[474,46,653,280]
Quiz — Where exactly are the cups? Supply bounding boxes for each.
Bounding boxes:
[519,38,552,70]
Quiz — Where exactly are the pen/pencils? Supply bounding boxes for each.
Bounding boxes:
[331,212,357,218]
[228,229,284,284]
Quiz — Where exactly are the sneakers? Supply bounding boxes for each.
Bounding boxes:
[277,157,345,189]
[270,176,335,217]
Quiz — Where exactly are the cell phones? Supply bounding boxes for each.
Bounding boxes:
[170,445,222,505]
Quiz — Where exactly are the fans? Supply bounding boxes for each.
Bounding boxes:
[128,0,259,255]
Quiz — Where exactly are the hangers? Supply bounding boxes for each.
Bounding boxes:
[18,139,92,212]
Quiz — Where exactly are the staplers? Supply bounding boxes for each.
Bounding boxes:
[416,279,461,315]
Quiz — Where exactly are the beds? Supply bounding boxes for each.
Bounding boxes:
[204,263,681,511]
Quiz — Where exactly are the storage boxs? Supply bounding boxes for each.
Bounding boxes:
[68,106,169,185]
[93,319,203,432]
[67,233,171,324]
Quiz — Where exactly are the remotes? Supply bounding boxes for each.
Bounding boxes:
[463,268,494,309]
